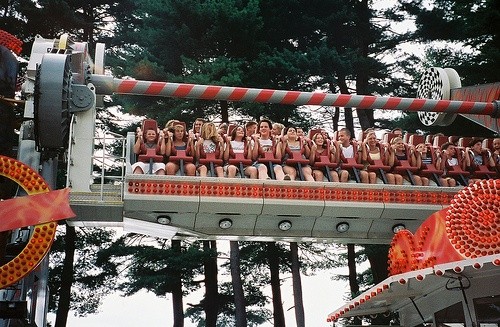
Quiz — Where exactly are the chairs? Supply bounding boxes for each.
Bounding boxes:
[137,119,500,189]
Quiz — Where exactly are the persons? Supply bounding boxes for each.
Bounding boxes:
[131,128,165,175]
[361,132,395,184]
[196,122,224,177]
[165,124,196,176]
[388,137,423,185]
[281,126,315,182]
[466,139,497,180]
[415,143,449,187]
[136,118,405,146]
[224,125,257,179]
[441,142,474,187]
[309,133,340,182]
[251,119,291,181]
[492,138,500,179]
[335,128,369,184]
